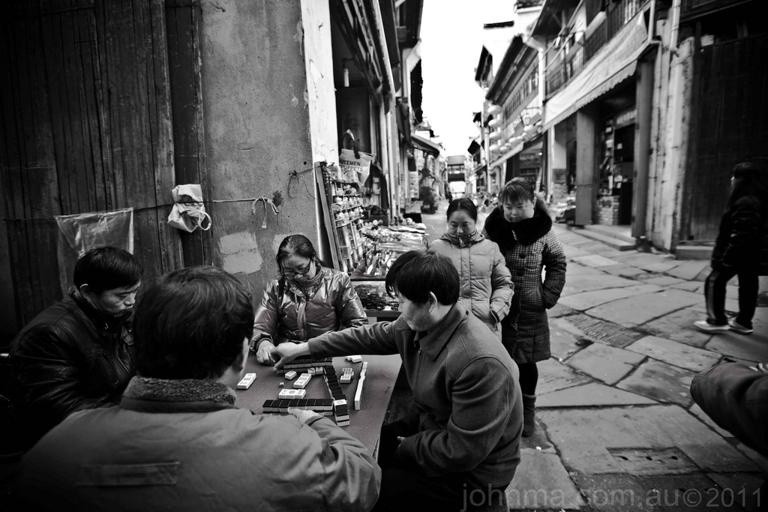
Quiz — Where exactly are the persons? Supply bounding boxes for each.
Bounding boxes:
[6,264,383,511]
[479,177,567,440]
[7,245,137,408]
[248,232,368,366]
[421,198,516,345]
[267,251,525,511]
[694,159,766,337]
[690,354,767,467]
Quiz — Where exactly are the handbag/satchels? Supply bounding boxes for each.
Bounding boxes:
[167,184,212,233]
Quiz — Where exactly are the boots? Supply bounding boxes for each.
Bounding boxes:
[522,394,537,437]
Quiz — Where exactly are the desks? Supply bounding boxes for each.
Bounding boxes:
[224,347,407,476]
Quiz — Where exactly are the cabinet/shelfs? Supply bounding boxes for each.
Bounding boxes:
[315,159,433,326]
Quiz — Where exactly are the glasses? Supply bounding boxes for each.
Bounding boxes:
[280,258,312,280]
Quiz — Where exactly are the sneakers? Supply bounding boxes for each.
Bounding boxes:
[725,311,754,334]
[694,320,731,335]
